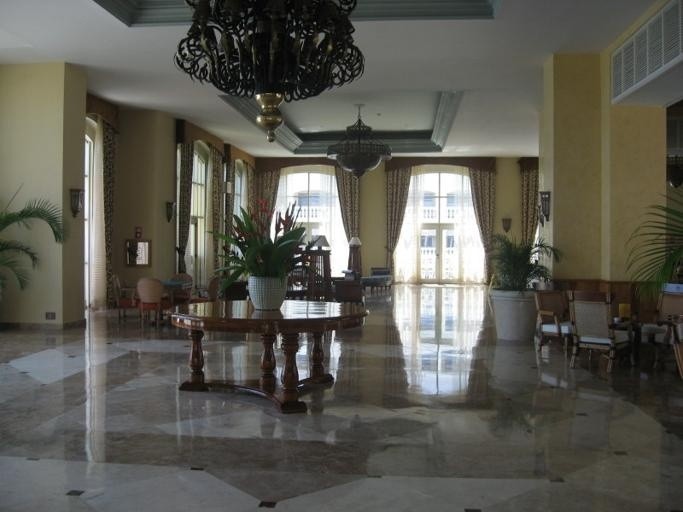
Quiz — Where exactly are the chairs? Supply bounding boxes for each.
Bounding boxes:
[103,266,396,331]
[530,286,682,381]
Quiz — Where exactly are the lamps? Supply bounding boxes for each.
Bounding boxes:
[69,188,83,217]
[502,218,511,232]
[534,205,544,227]
[171,0,365,143]
[165,199,176,223]
[309,233,330,251]
[348,236,361,270]
[321,101,393,180]
[538,189,550,222]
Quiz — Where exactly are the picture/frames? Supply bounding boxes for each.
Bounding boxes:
[123,238,152,268]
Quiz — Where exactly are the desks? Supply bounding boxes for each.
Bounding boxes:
[169,300,368,413]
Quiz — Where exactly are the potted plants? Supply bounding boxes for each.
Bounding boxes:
[479,230,561,343]
[215,195,319,312]
